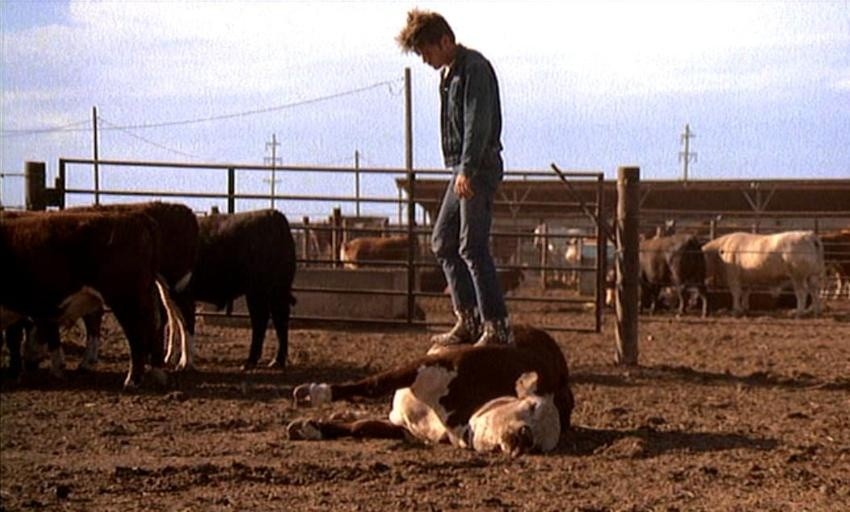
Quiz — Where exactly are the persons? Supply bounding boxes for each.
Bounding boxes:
[395,8,518,351]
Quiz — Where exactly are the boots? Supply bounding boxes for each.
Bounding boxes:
[472,312,515,347]
[430,307,481,346]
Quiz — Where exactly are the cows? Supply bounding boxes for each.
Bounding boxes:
[339,236,438,269]
[607,227,850,318]
[287,325,574,458]
[0,202,295,394]
